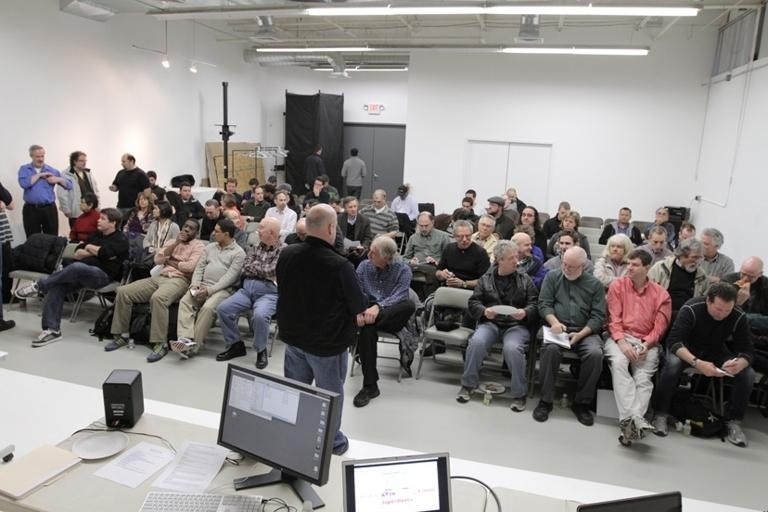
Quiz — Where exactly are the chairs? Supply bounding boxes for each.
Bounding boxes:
[478,325,533,404]
[652,341,720,442]
[70,240,126,333]
[724,349,768,424]
[236,305,283,362]
[351,284,417,389]
[532,320,598,416]
[8,237,65,326]
[413,284,476,391]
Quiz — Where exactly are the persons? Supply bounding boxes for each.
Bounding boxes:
[645,207,675,249]
[518,206,548,261]
[105,218,204,362]
[147,170,165,200]
[465,190,477,199]
[485,196,514,239]
[598,207,644,246]
[500,193,520,224]
[510,232,545,290]
[721,256,768,314]
[455,239,539,412]
[267,175,277,184]
[276,203,367,456]
[57,151,100,230]
[391,185,419,228]
[172,182,206,231]
[507,187,526,216]
[668,224,696,250]
[243,187,270,222]
[223,209,248,251]
[697,228,735,278]
[533,245,606,426]
[419,220,490,356]
[541,201,570,239]
[340,149,365,199]
[593,233,637,298]
[170,217,246,359]
[13,208,130,347]
[359,189,398,246]
[448,208,476,236]
[544,230,594,274]
[70,193,101,241]
[283,216,307,245]
[219,194,243,231]
[215,177,242,207]
[243,178,258,200]
[200,198,223,240]
[275,183,301,221]
[0,182,15,332]
[322,174,338,197]
[546,211,591,261]
[109,153,150,209]
[302,144,326,191]
[336,196,371,257]
[331,198,344,213]
[513,225,543,262]
[119,192,152,231]
[17,146,73,240]
[605,249,672,445]
[645,239,711,315]
[304,176,329,205]
[303,199,318,216]
[260,184,276,207]
[651,280,755,448]
[635,225,671,268]
[404,211,449,301]
[351,235,415,408]
[470,214,498,264]
[462,197,479,222]
[265,191,298,233]
[216,218,288,369]
[143,201,181,268]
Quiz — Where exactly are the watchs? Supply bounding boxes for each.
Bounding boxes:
[462,279,467,288]
[690,356,698,366]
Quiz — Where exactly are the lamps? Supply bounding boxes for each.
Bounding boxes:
[304,6,707,23]
[495,43,658,62]
[159,4,172,74]
[311,62,411,82]
[189,0,205,83]
[255,49,376,58]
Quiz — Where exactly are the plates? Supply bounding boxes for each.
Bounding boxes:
[479,381,506,395]
[71,431,127,459]
[490,304,517,316]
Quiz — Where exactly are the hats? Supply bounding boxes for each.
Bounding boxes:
[488,196,506,207]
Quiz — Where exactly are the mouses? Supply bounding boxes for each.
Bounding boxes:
[136,492,266,511]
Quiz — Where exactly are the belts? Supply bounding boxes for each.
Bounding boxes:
[26,202,54,208]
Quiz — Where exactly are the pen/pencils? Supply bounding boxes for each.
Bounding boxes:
[724,352,744,371]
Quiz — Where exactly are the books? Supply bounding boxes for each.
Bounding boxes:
[543,325,571,350]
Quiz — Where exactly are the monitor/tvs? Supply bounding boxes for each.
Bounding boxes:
[342,452,452,512]
[576,492,684,511]
[215,362,340,510]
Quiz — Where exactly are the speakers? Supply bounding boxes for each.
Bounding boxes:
[664,205,691,242]
[102,369,144,428]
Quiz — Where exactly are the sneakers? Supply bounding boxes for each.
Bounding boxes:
[181,344,199,361]
[510,398,525,412]
[650,414,669,437]
[726,420,748,447]
[455,388,471,402]
[0,318,16,331]
[147,345,168,362]
[31,332,63,346]
[618,416,636,446]
[632,416,654,439]
[104,337,129,351]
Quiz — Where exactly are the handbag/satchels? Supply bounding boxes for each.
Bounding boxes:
[91,303,114,339]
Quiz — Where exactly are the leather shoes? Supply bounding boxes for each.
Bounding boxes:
[353,385,381,408]
[216,342,248,362]
[419,346,446,359]
[256,349,268,369]
[532,400,553,422]
[572,405,594,426]
